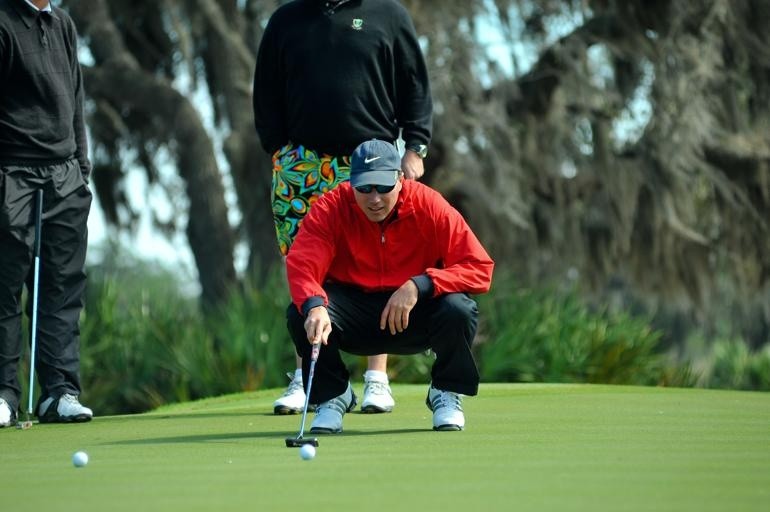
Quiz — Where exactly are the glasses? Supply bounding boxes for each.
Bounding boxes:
[355,185,395,193]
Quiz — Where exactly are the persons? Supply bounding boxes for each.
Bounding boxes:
[283,138,495,436]
[252,0,432,422]
[0,1,93,426]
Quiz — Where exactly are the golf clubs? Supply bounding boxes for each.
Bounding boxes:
[285,339,321,446]
[19,189,43,430]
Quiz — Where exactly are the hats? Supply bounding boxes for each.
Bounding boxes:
[350,138,401,188]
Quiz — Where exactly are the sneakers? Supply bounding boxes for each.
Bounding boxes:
[0,398,15,428]
[426,384,465,431]
[310,380,357,433]
[273,372,307,415]
[361,375,395,412]
[39,394,93,423]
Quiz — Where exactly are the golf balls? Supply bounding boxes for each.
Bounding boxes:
[73,451,88,467]
[301,443,315,459]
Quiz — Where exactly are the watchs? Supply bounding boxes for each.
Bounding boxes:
[404,140,429,160]
